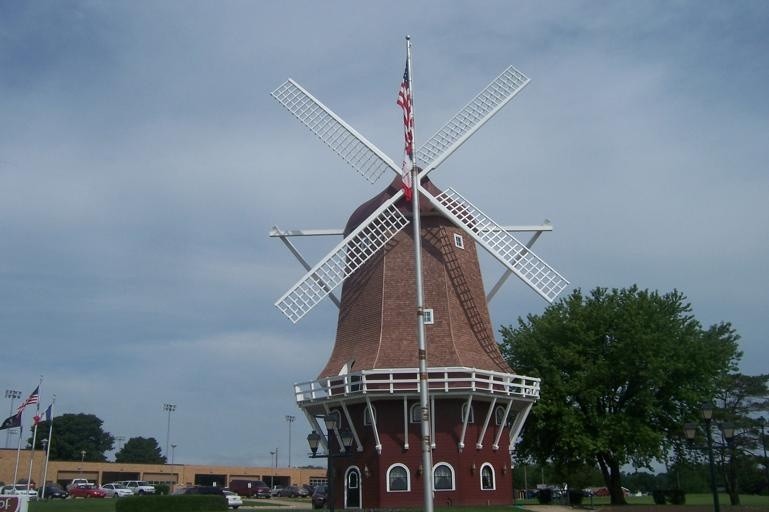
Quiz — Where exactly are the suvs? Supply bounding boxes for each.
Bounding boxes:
[69,479,155,497]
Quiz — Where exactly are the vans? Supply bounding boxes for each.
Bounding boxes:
[229,480,272,498]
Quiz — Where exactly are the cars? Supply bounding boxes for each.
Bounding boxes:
[38,486,68,498]
[274,484,327,508]
[3,485,38,499]
[522,486,628,498]
[172,485,243,509]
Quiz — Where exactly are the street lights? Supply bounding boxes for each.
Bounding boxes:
[41,438,47,486]
[270,451,275,497]
[162,401,175,464]
[170,442,177,463]
[4,389,21,448]
[285,415,295,468]
[79,451,85,476]
[682,406,734,511]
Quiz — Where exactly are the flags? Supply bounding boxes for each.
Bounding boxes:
[15,383,39,418]
[395,53,416,202]
[31,403,52,426]
[0,409,22,430]
